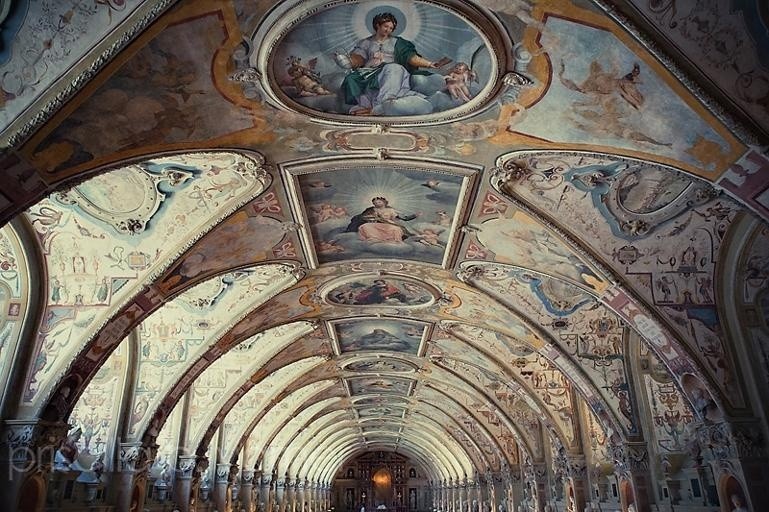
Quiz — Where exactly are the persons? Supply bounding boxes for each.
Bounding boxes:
[333,13,441,115]
[317,202,334,222]
[420,229,440,247]
[311,178,330,189]
[446,61,476,101]
[349,196,424,245]
[287,60,331,98]
[316,240,336,254]
[373,280,401,302]
[318,207,346,221]
